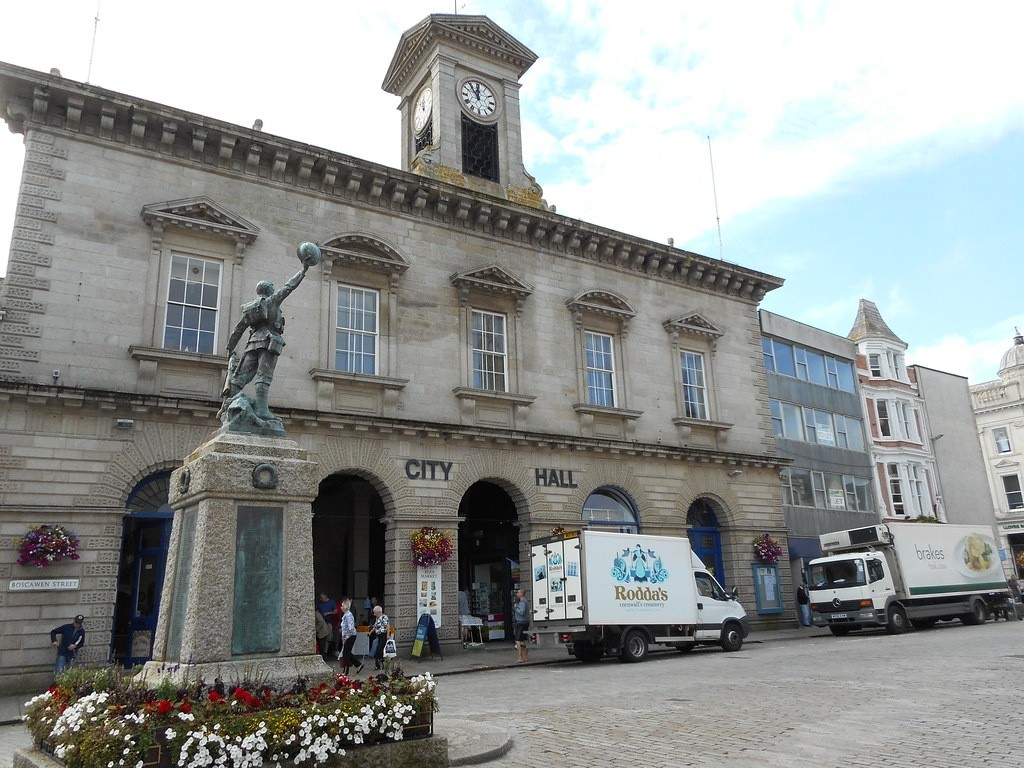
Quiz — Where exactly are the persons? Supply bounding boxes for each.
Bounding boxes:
[369,597,390,670]
[459,591,471,642]
[797,582,812,627]
[512,589,530,663]
[1007,574,1023,603]
[214,255,311,422]
[315,592,364,674]
[50,615,85,674]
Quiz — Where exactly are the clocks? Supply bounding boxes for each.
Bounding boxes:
[461,80,496,116]
[411,85,432,134]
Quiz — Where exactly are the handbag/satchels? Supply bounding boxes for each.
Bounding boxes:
[340,657,353,668]
[383,639,397,658]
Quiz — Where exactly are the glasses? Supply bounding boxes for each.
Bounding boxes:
[75,621,81,624]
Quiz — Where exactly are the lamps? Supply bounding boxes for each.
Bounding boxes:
[729,470,742,475]
[116,419,135,428]
[931,434,943,440]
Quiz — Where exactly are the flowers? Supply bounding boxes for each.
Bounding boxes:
[15,524,82,568]
[552,527,568,536]
[407,528,452,566]
[754,535,783,563]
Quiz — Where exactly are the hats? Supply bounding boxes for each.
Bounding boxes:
[76,614,84,622]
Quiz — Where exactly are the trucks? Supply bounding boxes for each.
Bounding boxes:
[800,521,1013,636]
[528,529,750,663]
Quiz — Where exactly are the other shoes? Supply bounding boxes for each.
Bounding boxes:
[357,665,364,673]
[517,659,525,663]
[803,624,811,627]
[372,667,380,671]
[524,658,528,661]
